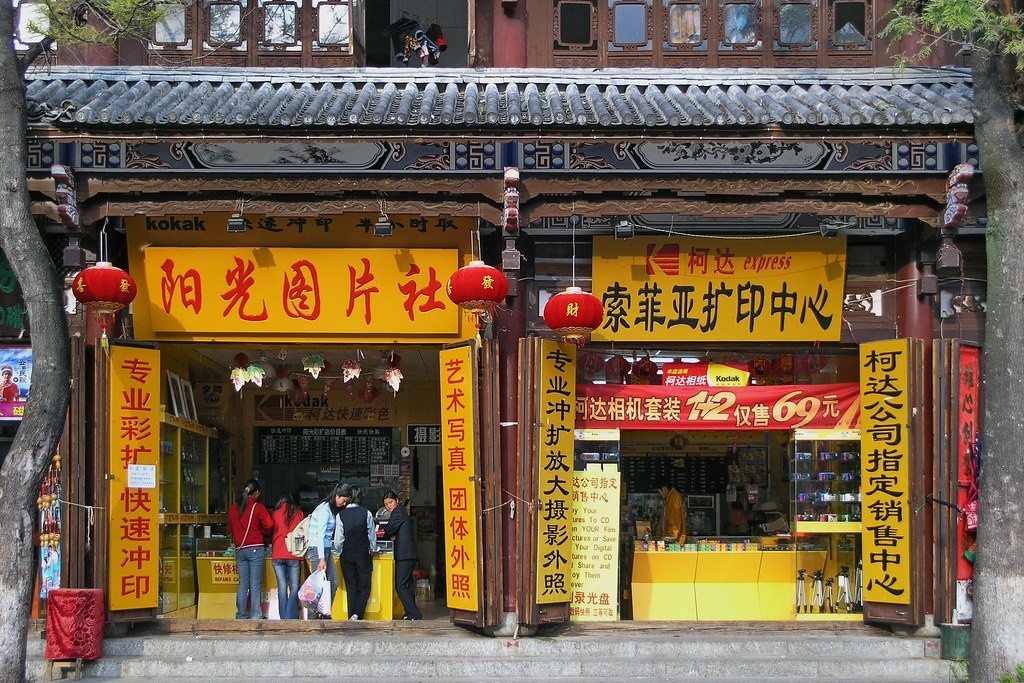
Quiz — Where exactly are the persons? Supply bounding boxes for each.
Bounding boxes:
[271,494,306,621]
[0,365,19,404]
[382,492,423,620]
[334,484,377,620]
[306,482,353,620]
[651,476,688,543]
[229,479,274,620]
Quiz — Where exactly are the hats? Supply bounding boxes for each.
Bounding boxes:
[1,365,13,376]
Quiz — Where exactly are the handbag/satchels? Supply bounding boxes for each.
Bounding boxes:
[313,569,332,617]
[298,569,324,609]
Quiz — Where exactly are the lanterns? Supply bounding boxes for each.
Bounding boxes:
[447,260,510,349]
[543,287,605,348]
[72,261,138,350]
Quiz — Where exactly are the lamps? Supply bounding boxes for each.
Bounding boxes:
[269,348,296,392]
[258,351,277,378]
[226,214,247,233]
[373,350,390,379]
[373,217,393,237]
[819,215,839,239]
[614,216,635,240]
[501,0,518,15]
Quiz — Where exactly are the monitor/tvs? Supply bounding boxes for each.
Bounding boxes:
[377,518,389,529]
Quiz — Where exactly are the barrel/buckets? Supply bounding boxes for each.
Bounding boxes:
[939,622,971,660]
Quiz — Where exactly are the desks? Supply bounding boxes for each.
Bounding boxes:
[46,588,105,682]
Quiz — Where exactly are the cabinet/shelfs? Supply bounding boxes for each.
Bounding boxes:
[789,428,861,533]
[157,412,234,621]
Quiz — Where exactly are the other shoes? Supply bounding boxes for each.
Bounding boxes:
[400,615,410,620]
[322,614,331,619]
[347,612,359,621]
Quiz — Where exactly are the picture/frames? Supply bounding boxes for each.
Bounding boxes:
[180,378,199,422]
[166,370,187,418]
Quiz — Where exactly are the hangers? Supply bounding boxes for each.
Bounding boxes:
[382,11,439,52]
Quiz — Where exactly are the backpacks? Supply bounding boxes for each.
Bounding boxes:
[284,502,332,558]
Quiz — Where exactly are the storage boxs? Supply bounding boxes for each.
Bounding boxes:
[633,540,758,551]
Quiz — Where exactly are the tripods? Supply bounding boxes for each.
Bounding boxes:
[796,555,862,613]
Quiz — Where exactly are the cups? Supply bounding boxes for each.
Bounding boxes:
[204,526,211,538]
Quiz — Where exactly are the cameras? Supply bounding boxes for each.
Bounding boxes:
[791,452,860,522]
